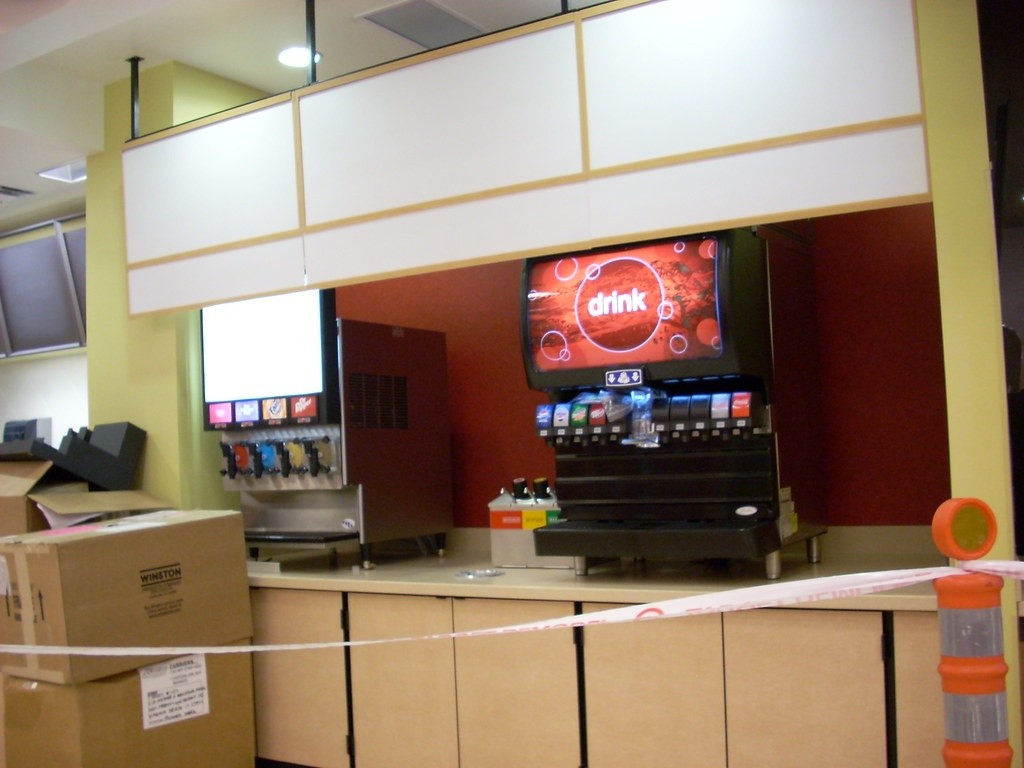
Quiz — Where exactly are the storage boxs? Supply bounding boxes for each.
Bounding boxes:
[0,458,255,768]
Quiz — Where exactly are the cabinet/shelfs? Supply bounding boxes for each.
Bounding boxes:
[248,555,937,768]
